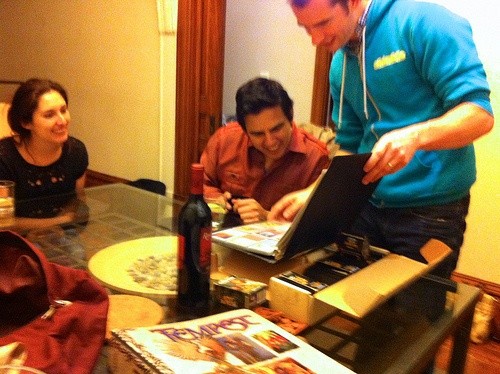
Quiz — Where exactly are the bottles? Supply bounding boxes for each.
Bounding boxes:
[177,164,212,304]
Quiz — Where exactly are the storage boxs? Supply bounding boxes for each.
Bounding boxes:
[265,236,452,329]
[213,274,267,310]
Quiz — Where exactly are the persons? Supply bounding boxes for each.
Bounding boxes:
[0,79,89,236]
[200,77,331,225]
[267,0,495,374]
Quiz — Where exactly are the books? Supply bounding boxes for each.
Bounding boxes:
[212,153,383,264]
[110,308,357,374]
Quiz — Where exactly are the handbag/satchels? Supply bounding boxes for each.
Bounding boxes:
[0,229,110,374]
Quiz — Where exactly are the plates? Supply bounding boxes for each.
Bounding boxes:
[87,233,186,301]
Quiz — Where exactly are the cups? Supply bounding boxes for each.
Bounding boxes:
[0,180,17,227]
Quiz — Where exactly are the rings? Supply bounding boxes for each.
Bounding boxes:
[387,163,392,167]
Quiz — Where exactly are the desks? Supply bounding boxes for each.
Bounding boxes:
[0,184,483,374]
